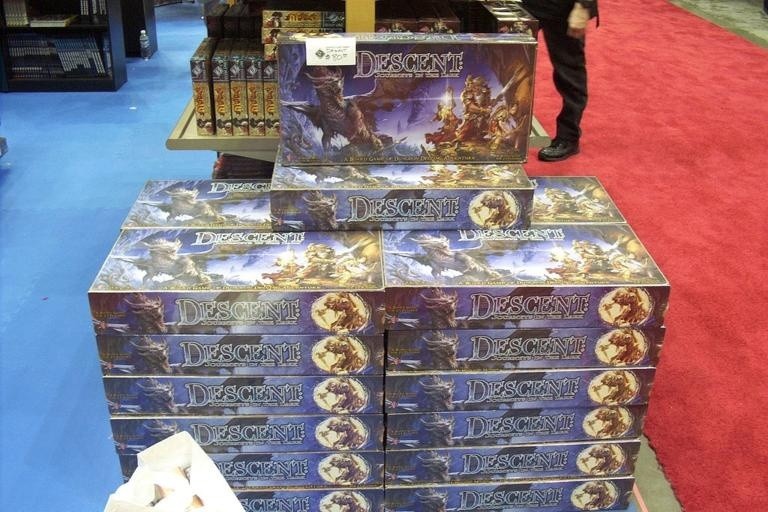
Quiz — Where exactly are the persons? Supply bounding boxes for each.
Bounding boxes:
[516,0,601,162]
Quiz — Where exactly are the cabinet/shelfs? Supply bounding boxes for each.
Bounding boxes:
[0,0,158,92]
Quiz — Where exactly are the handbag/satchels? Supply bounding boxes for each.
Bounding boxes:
[104,430,246,512]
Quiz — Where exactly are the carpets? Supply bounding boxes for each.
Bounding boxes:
[481,0,768,511]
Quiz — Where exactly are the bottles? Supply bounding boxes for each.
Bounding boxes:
[140,30,151,59]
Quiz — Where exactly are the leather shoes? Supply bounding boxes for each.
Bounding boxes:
[538,137,579,161]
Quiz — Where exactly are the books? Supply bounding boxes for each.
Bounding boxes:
[1,1,111,80]
